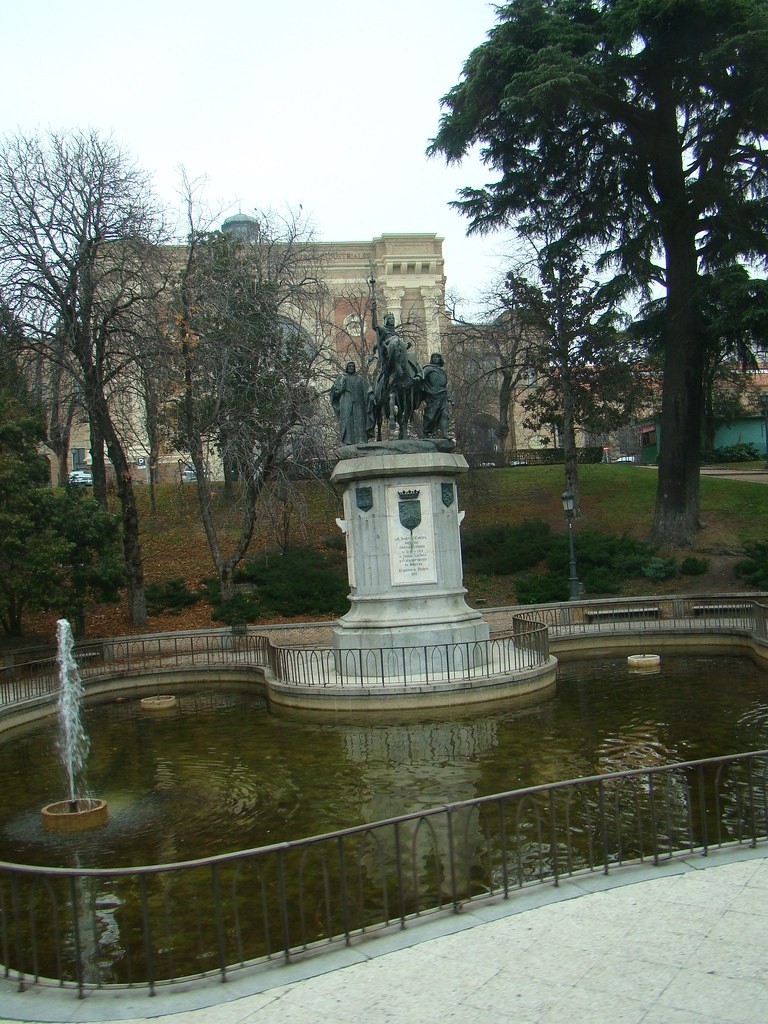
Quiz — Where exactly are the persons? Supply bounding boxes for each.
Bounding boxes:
[411,352,453,440]
[330,361,376,445]
[372,302,412,372]
